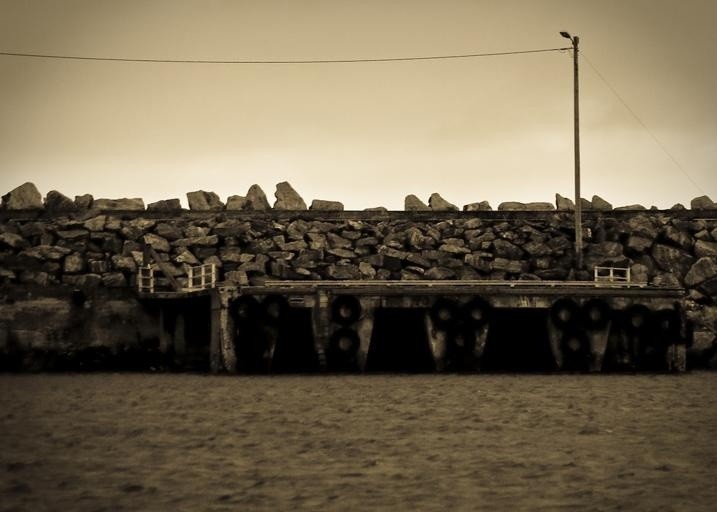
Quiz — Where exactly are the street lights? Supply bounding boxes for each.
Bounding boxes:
[558,29,582,254]
